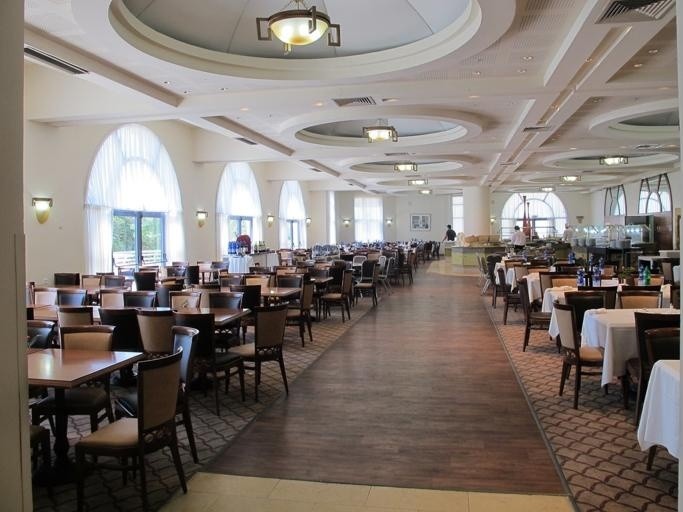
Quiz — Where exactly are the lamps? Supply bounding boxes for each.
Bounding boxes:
[386,218,392,226]
[29,196,52,224]
[416,190,432,195]
[196,210,208,227]
[392,162,417,172]
[407,178,428,185]
[266,215,274,228]
[305,218,311,227]
[362,118,398,144]
[342,219,351,228]
[559,175,583,184]
[598,154,628,166]
[254,0,342,55]
[537,185,558,193]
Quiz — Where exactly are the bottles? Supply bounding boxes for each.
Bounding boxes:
[252,240,265,253]
[502,249,652,286]
[226,241,248,254]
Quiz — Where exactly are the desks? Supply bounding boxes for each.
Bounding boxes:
[634,357,679,468]
[23,344,146,487]
[476,239,680,312]
[578,304,680,411]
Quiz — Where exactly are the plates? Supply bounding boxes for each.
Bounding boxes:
[570,238,630,249]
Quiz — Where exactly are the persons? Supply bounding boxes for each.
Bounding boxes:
[442,225,456,242]
[563,223,573,244]
[511,226,527,254]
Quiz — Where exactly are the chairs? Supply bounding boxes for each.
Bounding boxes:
[172,311,247,420]
[55,323,118,464]
[222,300,291,403]
[516,276,563,354]
[637,326,679,473]
[619,309,679,413]
[73,345,190,512]
[26,237,440,352]
[24,395,56,512]
[111,324,202,482]
[550,298,630,411]
[496,267,527,326]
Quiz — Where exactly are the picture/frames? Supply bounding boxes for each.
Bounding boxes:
[408,213,431,232]
